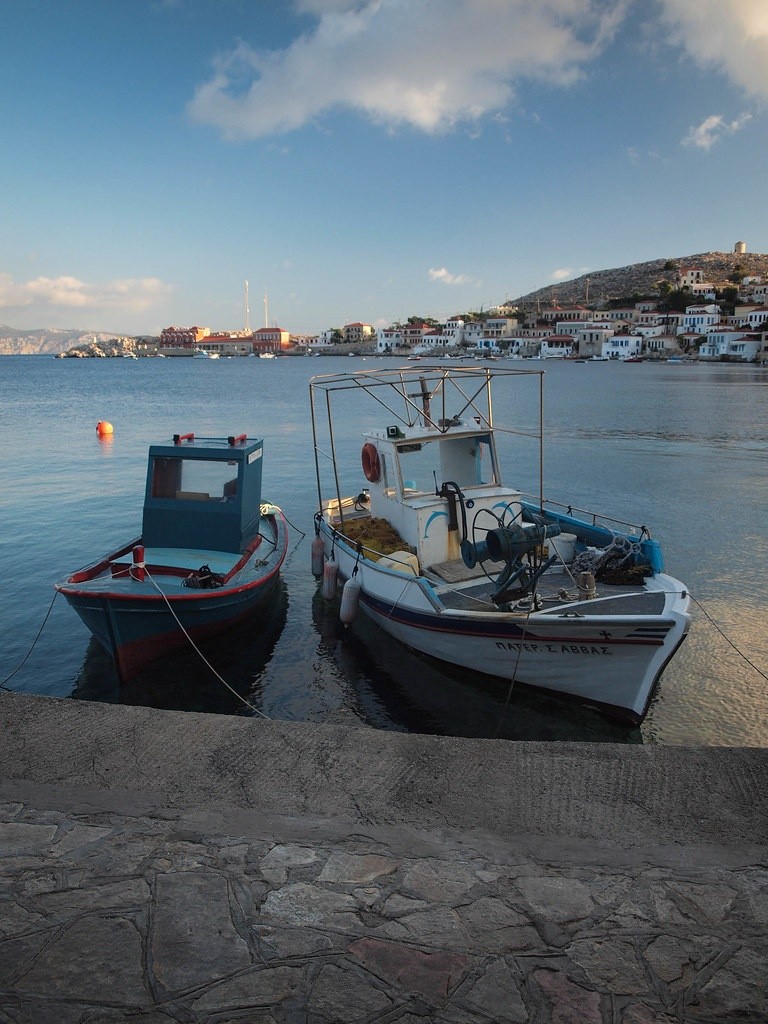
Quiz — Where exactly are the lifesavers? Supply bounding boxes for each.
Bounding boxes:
[361,443,380,482]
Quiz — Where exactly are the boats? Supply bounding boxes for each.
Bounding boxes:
[305,363,695,729]
[667,354,685,363]
[345,352,548,361]
[192,347,221,359]
[259,352,275,359]
[52,431,292,690]
[575,355,645,364]
